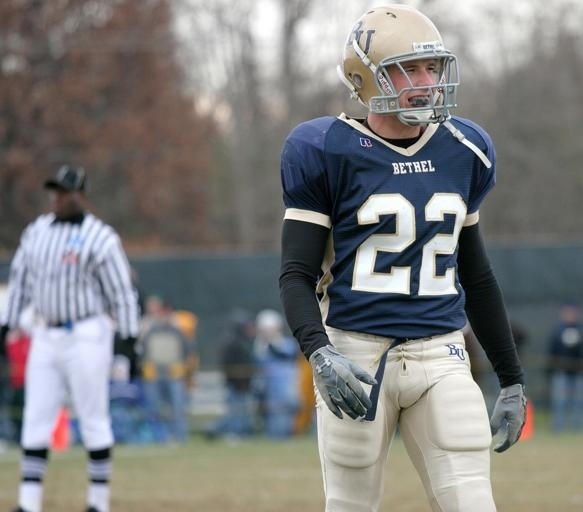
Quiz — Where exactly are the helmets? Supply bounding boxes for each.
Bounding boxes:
[337,4,460,127]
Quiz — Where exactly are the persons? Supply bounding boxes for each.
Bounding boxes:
[202,309,259,440]
[463,300,583,435]
[251,309,305,438]
[0,163,141,512]
[280,5,527,512]
[0,269,198,446]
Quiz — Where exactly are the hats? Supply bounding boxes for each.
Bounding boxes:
[46,165,86,191]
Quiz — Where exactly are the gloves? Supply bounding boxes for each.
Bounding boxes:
[308,344,377,421]
[115,337,138,381]
[490,383,526,454]
